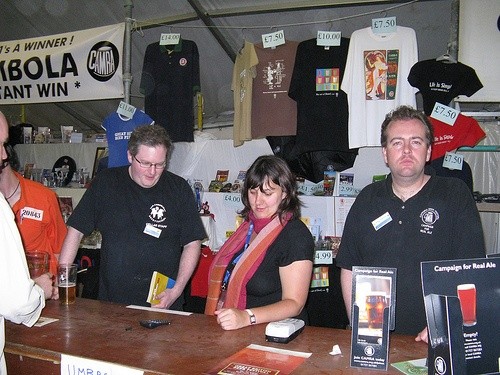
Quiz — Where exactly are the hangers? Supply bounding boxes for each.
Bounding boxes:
[436,53,457,63]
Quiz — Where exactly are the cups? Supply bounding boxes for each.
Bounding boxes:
[365,293,385,329]
[457,283,477,326]
[42,128,50,144]
[61,126,74,143]
[25,250,49,279]
[24,127,32,144]
[57,264,77,305]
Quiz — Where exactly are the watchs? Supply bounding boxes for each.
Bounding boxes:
[244,309,256,325]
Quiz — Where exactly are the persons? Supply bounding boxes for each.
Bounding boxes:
[0,110,54,375]
[51,124,210,310]
[334,104,487,344]
[0,141,68,300]
[205,155,316,330]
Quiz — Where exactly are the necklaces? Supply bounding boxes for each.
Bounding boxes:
[5,181,20,200]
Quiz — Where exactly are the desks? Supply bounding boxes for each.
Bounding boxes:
[4,294,427,375]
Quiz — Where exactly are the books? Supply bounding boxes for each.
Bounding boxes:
[146,271,177,304]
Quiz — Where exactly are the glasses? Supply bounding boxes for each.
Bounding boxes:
[133,155,167,169]
